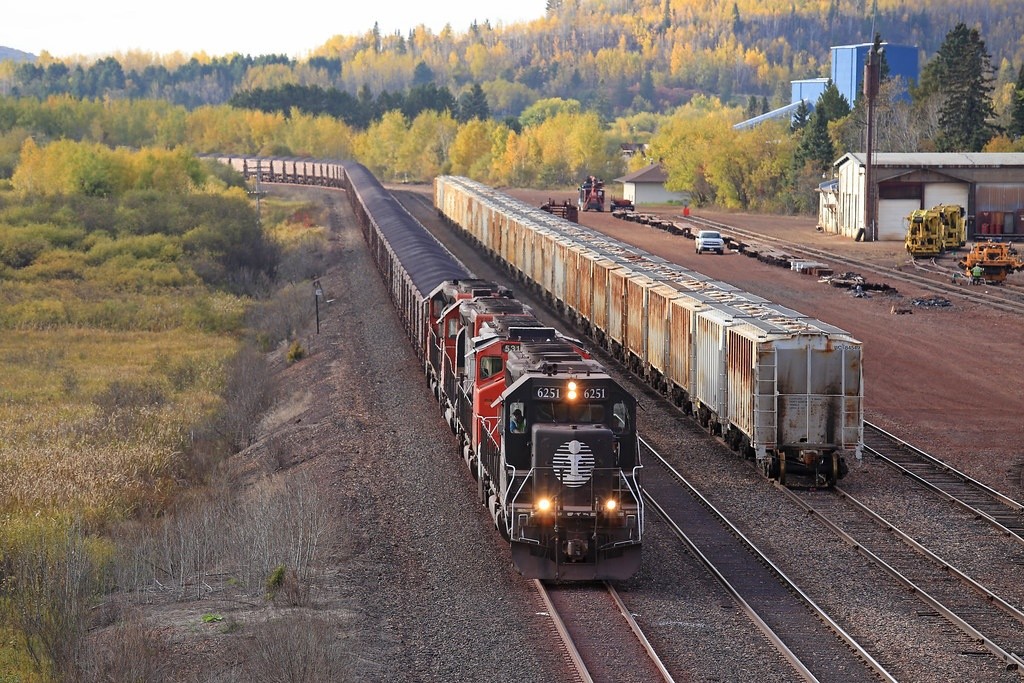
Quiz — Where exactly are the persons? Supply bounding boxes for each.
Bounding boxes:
[510,409,526,433]
[970,263,984,285]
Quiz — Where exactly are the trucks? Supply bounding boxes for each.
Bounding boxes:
[577,178,605,212]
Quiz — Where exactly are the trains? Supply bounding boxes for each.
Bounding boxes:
[432,173,864,491]
[198,155,644,583]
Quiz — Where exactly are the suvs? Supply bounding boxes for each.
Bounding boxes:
[695,231,724,255]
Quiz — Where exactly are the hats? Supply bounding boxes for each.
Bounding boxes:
[976,263,979,266]
